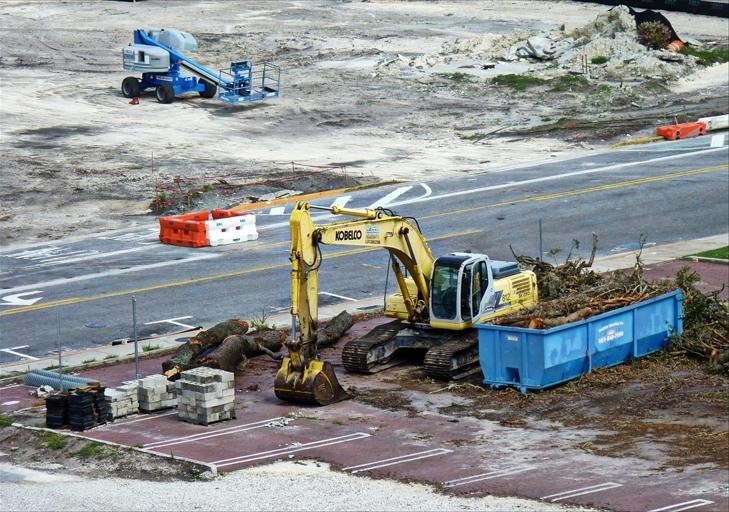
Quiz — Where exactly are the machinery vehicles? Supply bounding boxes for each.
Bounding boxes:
[272,199,540,407]
[120,23,284,106]
